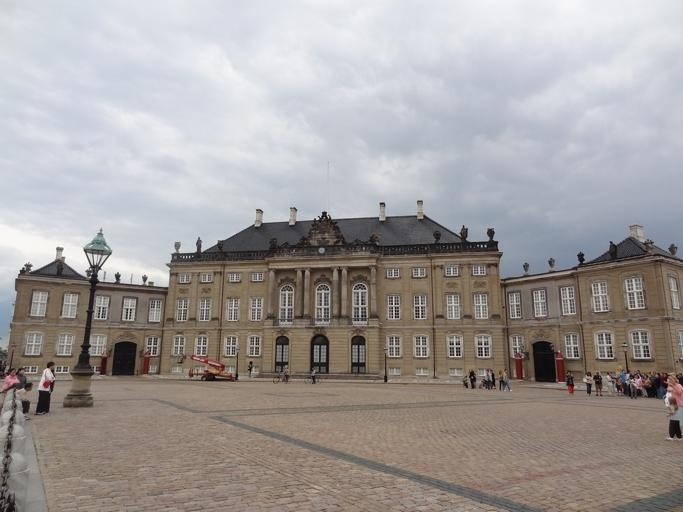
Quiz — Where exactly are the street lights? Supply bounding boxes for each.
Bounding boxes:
[622,342,630,374]
[63,228,113,407]
[235,347,240,379]
[384,346,388,382]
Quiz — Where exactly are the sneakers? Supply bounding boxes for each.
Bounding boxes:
[23,416,30,420]
[674,437,682,440]
[37,411,46,415]
[666,437,673,441]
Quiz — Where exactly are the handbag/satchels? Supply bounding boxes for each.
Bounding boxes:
[43,380,51,388]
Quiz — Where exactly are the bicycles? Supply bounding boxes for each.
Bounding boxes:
[305,375,322,383]
[273,370,290,384]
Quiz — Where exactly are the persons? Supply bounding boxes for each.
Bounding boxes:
[1,367,18,397]
[283,365,290,384]
[665,375,683,436]
[247,360,253,378]
[310,367,316,384]
[12,383,33,420]
[463,367,513,392]
[33,361,55,415]
[49,369,55,395]
[14,367,25,390]
[563,368,683,399]
[664,397,683,441]
